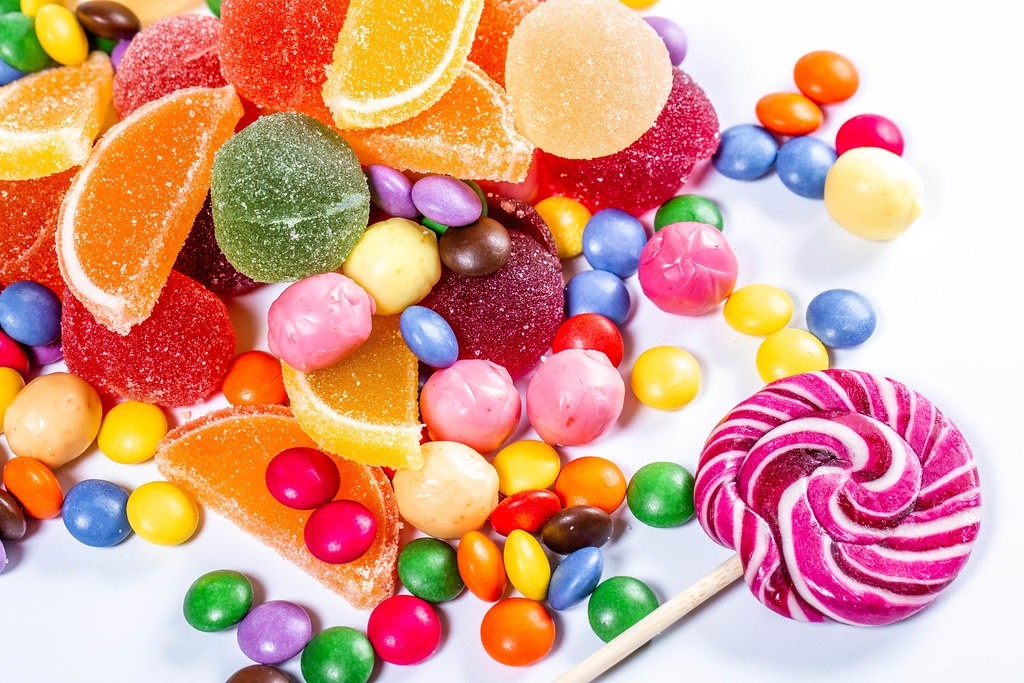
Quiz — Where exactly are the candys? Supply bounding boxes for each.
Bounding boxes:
[0,0,984,683]
